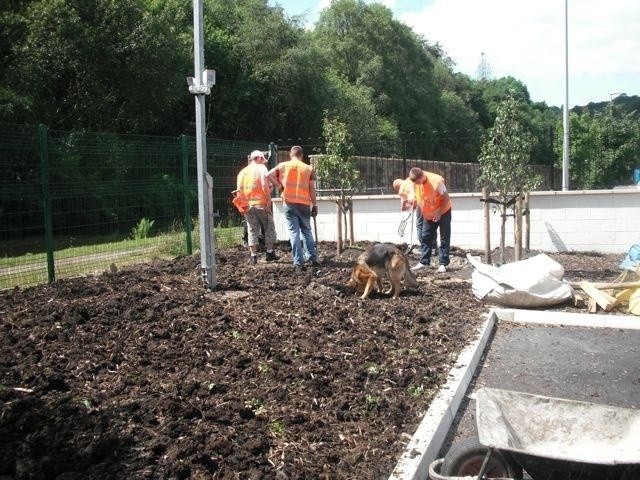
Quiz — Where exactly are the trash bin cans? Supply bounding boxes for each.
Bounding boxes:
[634,168,640,184]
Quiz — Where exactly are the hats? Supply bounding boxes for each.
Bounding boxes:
[251,150,268,164]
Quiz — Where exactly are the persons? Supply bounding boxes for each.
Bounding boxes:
[408,167,453,273]
[393,177,438,256]
[265,145,323,268]
[236,150,281,265]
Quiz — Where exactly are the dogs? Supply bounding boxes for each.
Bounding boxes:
[344,244,419,300]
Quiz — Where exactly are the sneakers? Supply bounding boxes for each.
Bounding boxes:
[435,265,448,273]
[431,248,437,255]
[265,251,284,263]
[411,262,430,270]
[250,255,257,265]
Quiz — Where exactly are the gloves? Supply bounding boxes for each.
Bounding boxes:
[311,205,318,217]
[278,185,284,193]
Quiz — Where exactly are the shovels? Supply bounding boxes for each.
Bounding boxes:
[406,210,414,253]
[313,212,321,265]
